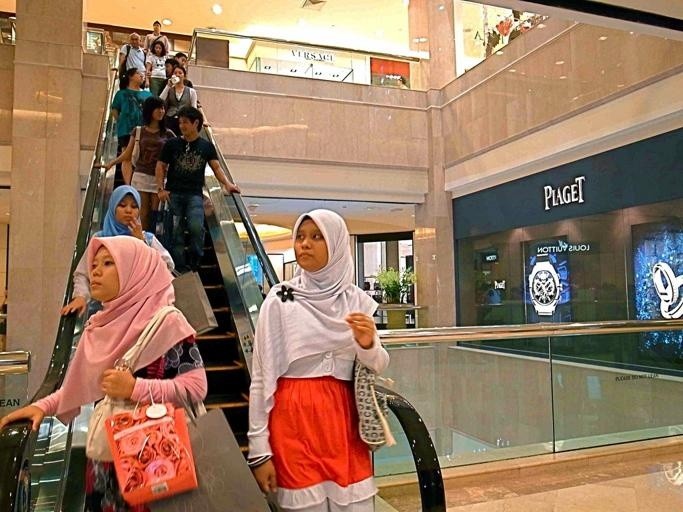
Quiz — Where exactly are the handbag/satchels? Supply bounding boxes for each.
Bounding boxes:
[166,268,218,335]
[86,306,178,462]
[147,383,269,511]
[132,126,141,168]
[118,63,129,89]
[148,200,181,247]
[106,380,198,510]
[354,362,397,452]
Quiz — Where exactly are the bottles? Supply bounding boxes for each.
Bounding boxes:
[171,76,180,84]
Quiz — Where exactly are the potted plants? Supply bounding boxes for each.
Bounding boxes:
[374,263,416,328]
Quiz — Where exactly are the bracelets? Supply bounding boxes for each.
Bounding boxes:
[248,455,271,468]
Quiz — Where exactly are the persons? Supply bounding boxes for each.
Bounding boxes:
[398,78,409,89]
[0,286,8,352]
[144,21,169,52]
[0,235,208,512]
[112,33,197,191]
[247,209,390,512]
[60,185,175,318]
[94,97,176,231]
[156,106,240,274]
